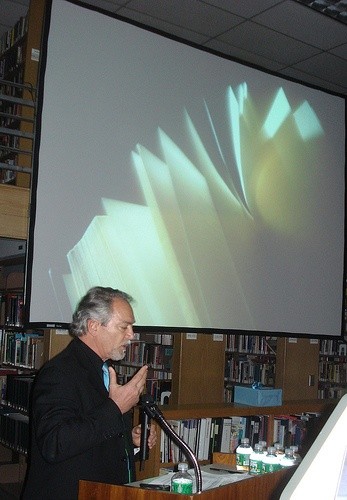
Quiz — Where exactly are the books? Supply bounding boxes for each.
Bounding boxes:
[95,331,347,470]
[0,14,28,184]
[0,294,45,450]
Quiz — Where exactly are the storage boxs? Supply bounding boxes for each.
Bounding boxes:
[235,385,282,407]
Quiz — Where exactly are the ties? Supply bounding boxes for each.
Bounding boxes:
[102,362,110,393]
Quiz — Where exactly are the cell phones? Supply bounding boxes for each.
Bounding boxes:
[139,483,170,491]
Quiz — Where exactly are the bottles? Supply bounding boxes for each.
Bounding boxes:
[259,441,268,457]
[274,443,285,463]
[171,463,193,495]
[290,446,302,467]
[262,447,282,475]
[280,449,298,469]
[250,444,266,476]
[236,438,253,471]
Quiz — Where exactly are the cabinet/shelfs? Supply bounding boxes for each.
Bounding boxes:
[0,0,44,187]
[319,335,347,399]
[108,333,226,426]
[132,398,338,481]
[0,271,73,464]
[224,334,320,403]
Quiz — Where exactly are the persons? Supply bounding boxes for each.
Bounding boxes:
[21,285,150,500]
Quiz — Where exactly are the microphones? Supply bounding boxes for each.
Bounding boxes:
[137,395,153,462]
